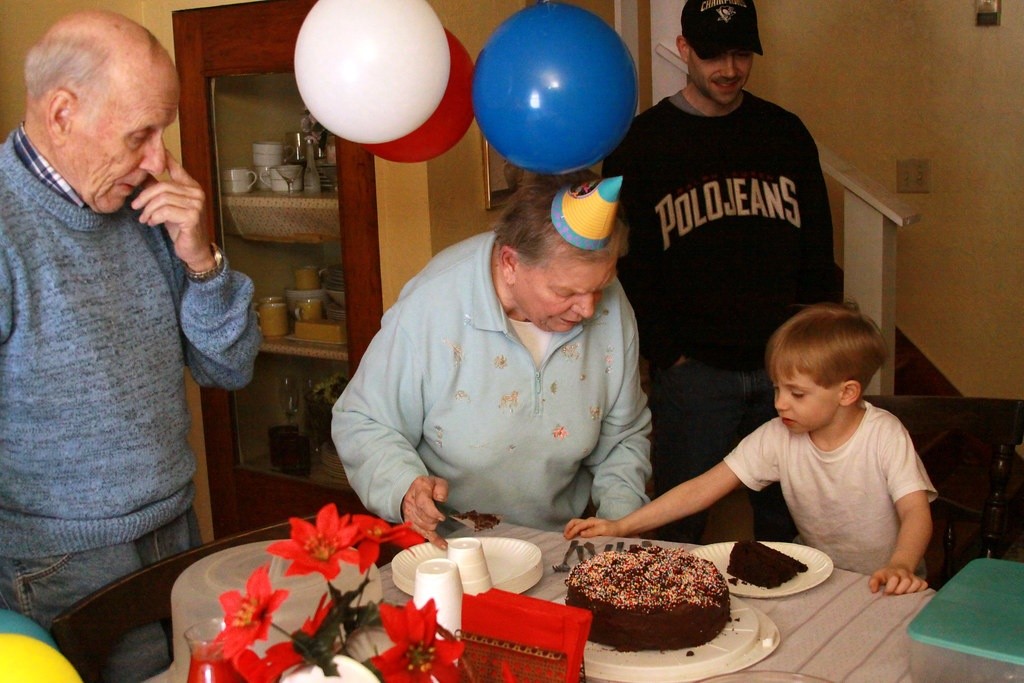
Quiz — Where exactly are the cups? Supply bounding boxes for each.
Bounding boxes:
[447,537,493,597]
[268,425,311,475]
[253,296,287,336]
[294,298,322,320]
[220,142,302,193]
[414,558,467,662]
[297,267,320,289]
[279,377,300,425]
[285,131,306,160]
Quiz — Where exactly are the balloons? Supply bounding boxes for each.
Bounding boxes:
[295,0,451,143]
[356,27,475,163]
[0,608,61,657]
[472,0,639,175]
[0,633,83,683]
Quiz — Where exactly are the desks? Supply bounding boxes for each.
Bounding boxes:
[150,518,941,683]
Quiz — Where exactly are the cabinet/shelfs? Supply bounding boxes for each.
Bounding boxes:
[171,0,384,537]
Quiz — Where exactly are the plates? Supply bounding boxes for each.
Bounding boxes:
[286,264,346,321]
[316,164,338,187]
[391,537,544,597]
[690,540,834,598]
[322,442,349,479]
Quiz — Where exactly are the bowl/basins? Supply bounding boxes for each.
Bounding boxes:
[695,667,835,683]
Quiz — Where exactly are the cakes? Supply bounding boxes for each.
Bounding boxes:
[728,539,808,587]
[565,544,731,652]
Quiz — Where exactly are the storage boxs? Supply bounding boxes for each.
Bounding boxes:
[905,558,1024,683]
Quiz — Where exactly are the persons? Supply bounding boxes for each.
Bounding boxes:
[602,0,836,544]
[563,301,938,596]
[0,9,263,683]
[331,167,652,551]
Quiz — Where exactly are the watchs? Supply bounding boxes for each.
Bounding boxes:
[184,242,224,284]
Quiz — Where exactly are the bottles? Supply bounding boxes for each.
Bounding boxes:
[304,140,321,197]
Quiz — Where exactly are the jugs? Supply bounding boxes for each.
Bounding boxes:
[184,617,245,683]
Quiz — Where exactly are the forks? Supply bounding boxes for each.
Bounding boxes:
[552,540,579,572]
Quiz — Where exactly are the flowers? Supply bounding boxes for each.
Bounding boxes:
[210,496,466,682]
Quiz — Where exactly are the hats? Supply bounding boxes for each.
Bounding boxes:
[550,172,623,251]
[681,0,763,60]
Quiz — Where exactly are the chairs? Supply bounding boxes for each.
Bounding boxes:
[863,395,1024,587]
[50,523,289,683]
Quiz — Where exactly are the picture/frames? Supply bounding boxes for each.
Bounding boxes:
[481,133,512,210]
